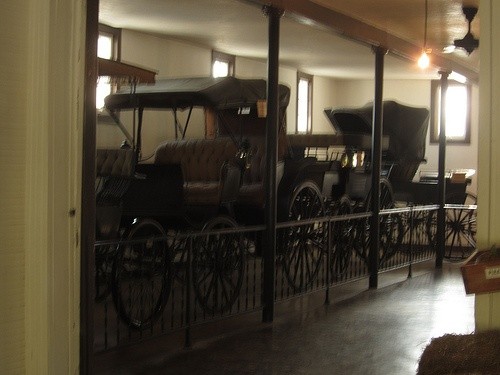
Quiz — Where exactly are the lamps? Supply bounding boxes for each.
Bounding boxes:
[416,0,435,71]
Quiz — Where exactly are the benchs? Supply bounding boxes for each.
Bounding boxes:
[94,136,271,239]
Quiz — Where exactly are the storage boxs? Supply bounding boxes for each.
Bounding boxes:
[459,247,500,296]
